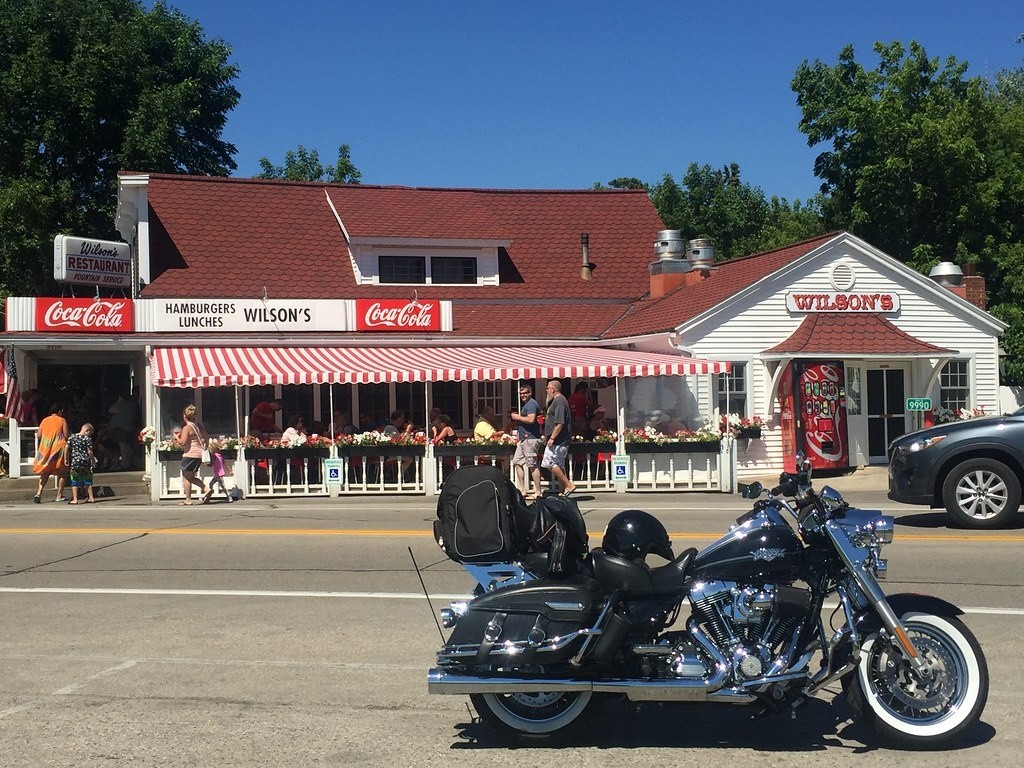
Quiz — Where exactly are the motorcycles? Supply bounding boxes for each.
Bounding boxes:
[407,447,989,750]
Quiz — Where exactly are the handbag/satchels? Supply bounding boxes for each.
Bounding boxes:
[436,465,529,563]
[201,450,211,464]
[524,491,589,554]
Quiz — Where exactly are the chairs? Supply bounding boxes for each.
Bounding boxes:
[249,449,616,485]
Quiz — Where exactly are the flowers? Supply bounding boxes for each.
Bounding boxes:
[934,404,990,422]
[138,425,156,443]
[721,414,765,438]
[158,425,717,452]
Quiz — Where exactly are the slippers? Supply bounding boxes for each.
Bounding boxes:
[55,495,68,502]
[85,500,95,503]
[34,495,41,503]
[200,489,212,498]
[178,500,192,506]
[68,501,77,504]
[558,485,576,496]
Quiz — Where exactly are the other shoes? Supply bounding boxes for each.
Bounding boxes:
[226,497,233,503]
[525,493,542,499]
[110,461,120,470]
[199,497,209,503]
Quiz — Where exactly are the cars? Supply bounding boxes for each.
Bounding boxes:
[888,403,1024,532]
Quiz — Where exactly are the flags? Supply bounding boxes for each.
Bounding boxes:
[5,345,24,423]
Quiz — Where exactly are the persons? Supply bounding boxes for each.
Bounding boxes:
[33,404,69,503]
[64,423,96,505]
[199,442,234,503]
[97,385,140,472]
[250,381,689,482]
[511,384,543,501]
[173,405,212,506]
[541,380,577,496]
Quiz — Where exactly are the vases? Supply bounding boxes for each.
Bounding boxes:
[932,418,957,426]
[145,437,153,450]
[738,430,760,440]
[158,437,720,462]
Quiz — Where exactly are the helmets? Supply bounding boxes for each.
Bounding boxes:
[603,511,674,562]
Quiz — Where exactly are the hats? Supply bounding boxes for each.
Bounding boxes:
[593,406,607,416]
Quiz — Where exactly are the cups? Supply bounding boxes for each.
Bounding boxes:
[537,415,544,424]
[312,434,318,439]
[418,432,424,436]
[512,411,518,414]
[174,432,180,440]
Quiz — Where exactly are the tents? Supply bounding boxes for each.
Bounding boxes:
[148,347,731,456]
